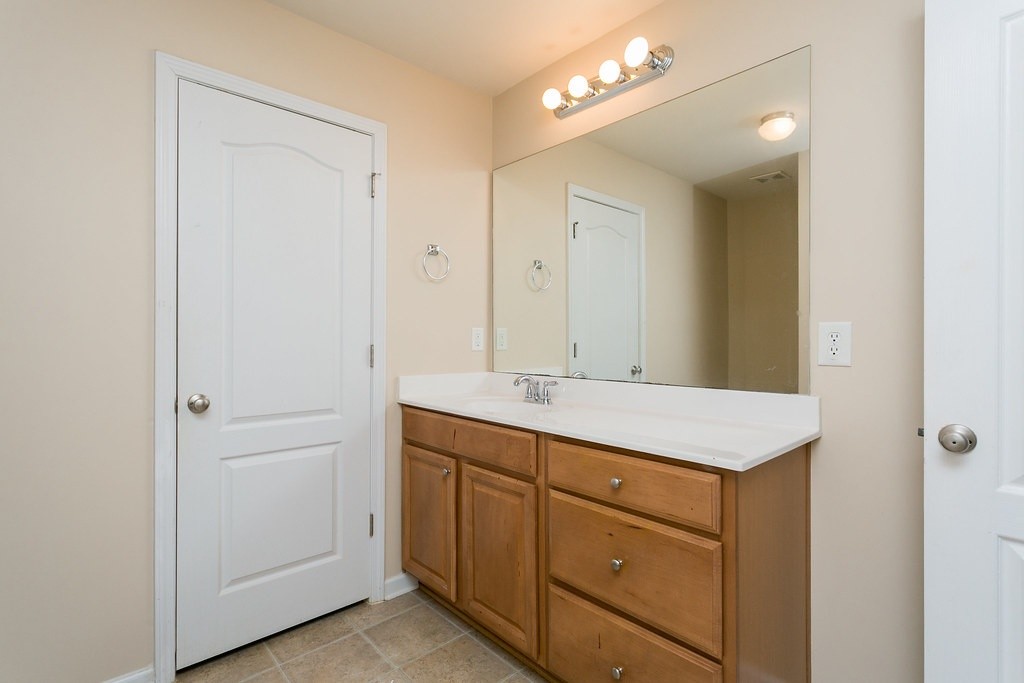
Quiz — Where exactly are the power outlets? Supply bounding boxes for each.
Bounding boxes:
[496,328,508,350]
[818,321,852,367]
[472,328,484,351]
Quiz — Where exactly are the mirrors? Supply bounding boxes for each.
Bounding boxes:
[492,44,811,395]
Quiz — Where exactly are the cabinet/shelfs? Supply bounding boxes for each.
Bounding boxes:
[401,405,811,683]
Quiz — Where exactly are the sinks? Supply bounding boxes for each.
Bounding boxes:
[450,398,563,425]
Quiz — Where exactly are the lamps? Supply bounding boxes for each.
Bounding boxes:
[757,111,796,141]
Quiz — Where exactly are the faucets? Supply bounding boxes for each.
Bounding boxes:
[570,371,589,379]
[513,375,559,405]
